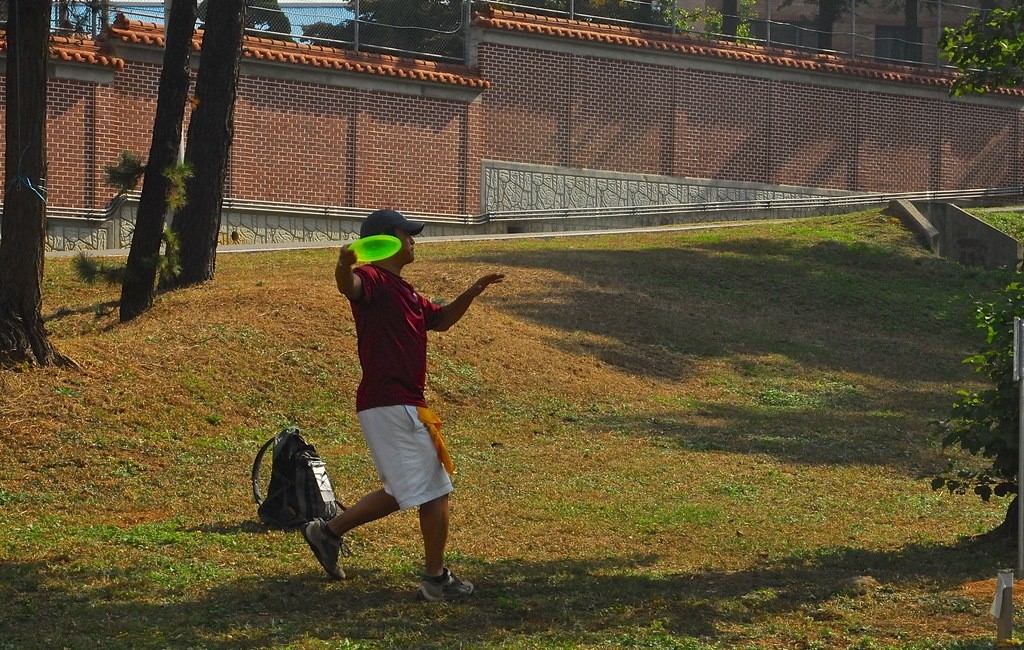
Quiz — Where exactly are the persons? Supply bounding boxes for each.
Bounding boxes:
[300,208,506,602]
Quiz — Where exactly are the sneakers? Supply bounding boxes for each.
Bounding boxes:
[301,520,347,579]
[416,567,474,602]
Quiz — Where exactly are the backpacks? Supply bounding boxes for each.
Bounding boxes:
[252,426,337,531]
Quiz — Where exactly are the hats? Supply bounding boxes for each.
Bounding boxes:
[361,209,425,238]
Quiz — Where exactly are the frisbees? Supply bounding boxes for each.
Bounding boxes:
[349,232,403,263]
